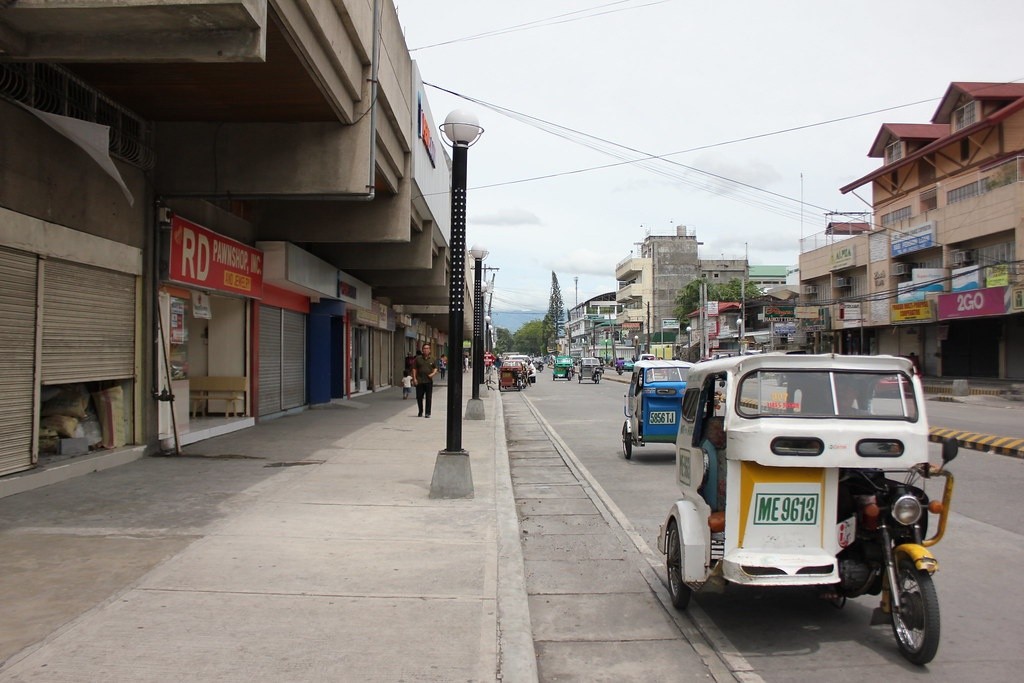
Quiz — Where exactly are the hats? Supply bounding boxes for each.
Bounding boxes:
[407,352,412,355]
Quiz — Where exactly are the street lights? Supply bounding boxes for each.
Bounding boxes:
[737,319,743,355]
[574,276,579,311]
[605,340,608,363]
[485,316,491,352]
[635,336,639,361]
[465,243,489,420]
[701,274,707,357]
[686,327,691,362]
[429,110,484,500]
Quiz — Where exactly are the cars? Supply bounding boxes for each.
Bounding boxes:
[873,356,924,397]
[505,355,537,383]
[772,350,807,386]
[639,354,655,361]
[544,351,560,364]
[615,360,635,372]
[571,352,581,362]
[573,360,582,373]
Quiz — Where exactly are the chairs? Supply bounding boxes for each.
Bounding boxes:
[703,415,727,542]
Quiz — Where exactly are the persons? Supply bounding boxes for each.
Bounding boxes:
[824,382,871,416]
[464,356,468,373]
[440,354,447,379]
[616,358,623,371]
[401,372,413,399]
[525,361,533,371]
[413,345,437,418]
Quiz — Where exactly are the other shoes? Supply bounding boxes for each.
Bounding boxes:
[418,413,422,417]
[403,396,407,400]
[425,414,429,418]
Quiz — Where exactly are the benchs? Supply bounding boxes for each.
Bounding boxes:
[187,375,249,418]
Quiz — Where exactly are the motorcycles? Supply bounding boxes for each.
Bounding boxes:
[657,352,959,665]
[552,356,575,380]
[621,360,725,459]
[578,358,605,384]
[537,362,544,373]
[498,360,528,391]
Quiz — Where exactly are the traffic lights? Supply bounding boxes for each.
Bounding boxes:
[591,339,595,345]
